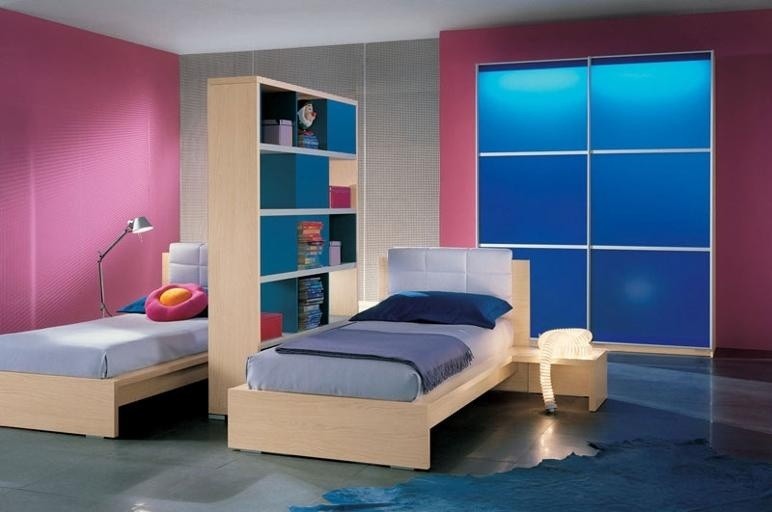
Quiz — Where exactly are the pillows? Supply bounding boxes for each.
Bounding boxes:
[115,292,209,317]
[347,290,513,329]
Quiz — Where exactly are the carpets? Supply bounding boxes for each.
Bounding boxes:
[281,436,772,511]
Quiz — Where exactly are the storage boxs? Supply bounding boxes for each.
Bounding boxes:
[329,186,351,209]
[260,120,293,146]
[329,240,342,265]
[261,311,283,342]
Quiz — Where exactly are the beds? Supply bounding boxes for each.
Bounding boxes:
[226,245,518,470]
[0,242,208,438]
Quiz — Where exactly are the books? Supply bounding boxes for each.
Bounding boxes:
[298,135,319,149]
[297,221,325,270]
[298,278,324,331]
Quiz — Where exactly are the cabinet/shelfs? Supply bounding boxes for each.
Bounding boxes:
[206,74,360,422]
[517,337,609,413]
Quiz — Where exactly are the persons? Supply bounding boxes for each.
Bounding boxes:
[297,100,317,136]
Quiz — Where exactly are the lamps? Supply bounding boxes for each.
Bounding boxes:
[97,216,156,317]
[537,327,594,416]
